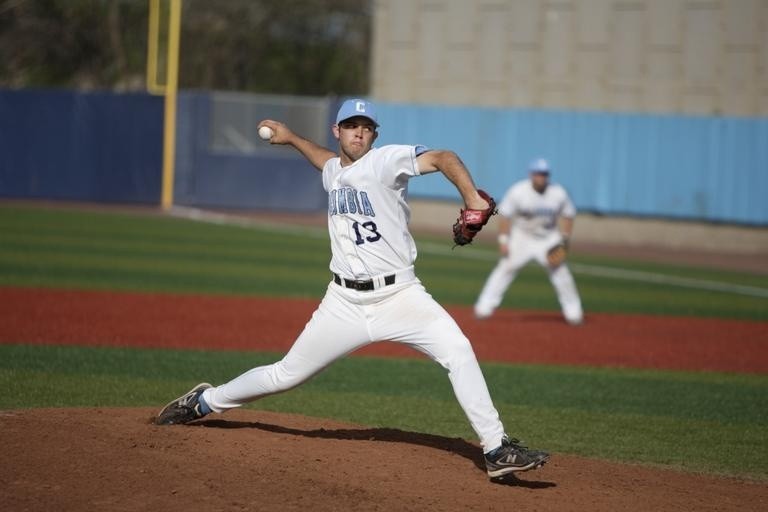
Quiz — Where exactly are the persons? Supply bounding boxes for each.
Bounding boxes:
[158,98,549,477]
[473,158,585,327]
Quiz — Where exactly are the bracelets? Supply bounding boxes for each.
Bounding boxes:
[497,231,509,245]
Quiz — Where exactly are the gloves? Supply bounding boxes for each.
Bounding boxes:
[548,234,570,269]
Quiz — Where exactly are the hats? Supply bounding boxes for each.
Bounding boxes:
[528,157,551,175]
[336,98,381,128]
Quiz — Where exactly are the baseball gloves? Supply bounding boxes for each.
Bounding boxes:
[548,247,566,267]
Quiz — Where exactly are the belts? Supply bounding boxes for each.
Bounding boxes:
[332,271,397,292]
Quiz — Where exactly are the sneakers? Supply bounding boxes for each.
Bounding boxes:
[484,441,551,480]
[156,381,214,427]
[567,314,592,333]
[470,312,491,326]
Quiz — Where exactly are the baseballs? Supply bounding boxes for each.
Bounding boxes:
[259,127,273,140]
